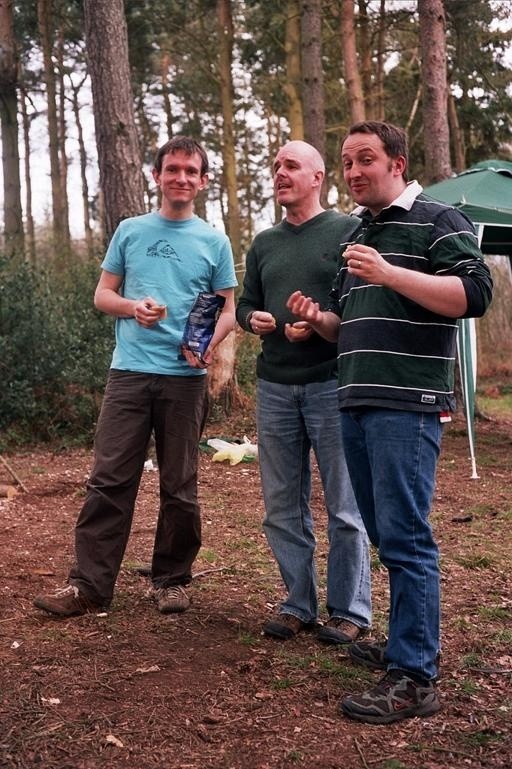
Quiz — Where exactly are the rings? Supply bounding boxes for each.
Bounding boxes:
[255,326,258,331]
[359,260,361,268]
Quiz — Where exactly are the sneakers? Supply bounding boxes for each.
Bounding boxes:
[33,584,92,617]
[348,639,392,670]
[155,583,193,612]
[264,613,302,640]
[317,617,360,644]
[344,669,441,724]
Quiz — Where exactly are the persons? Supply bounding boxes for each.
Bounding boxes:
[285,121,493,726]
[33,135,239,619]
[235,139,373,644]
